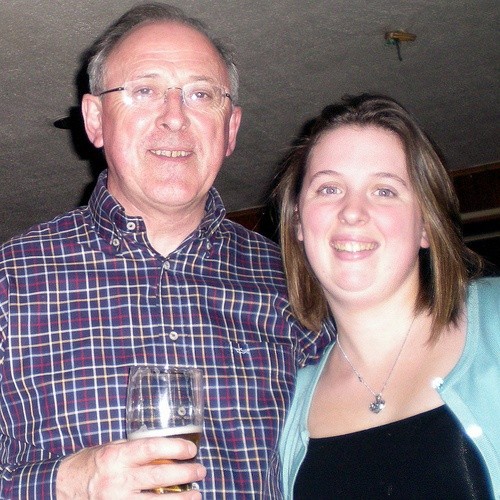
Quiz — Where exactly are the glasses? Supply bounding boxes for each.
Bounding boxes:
[95,78,233,113]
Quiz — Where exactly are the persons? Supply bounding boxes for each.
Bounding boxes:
[0,0,339,500]
[271,94,500,500]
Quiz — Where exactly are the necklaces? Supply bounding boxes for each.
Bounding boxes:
[336,314,416,413]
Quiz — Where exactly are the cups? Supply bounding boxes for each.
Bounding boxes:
[122,364,203,496]
